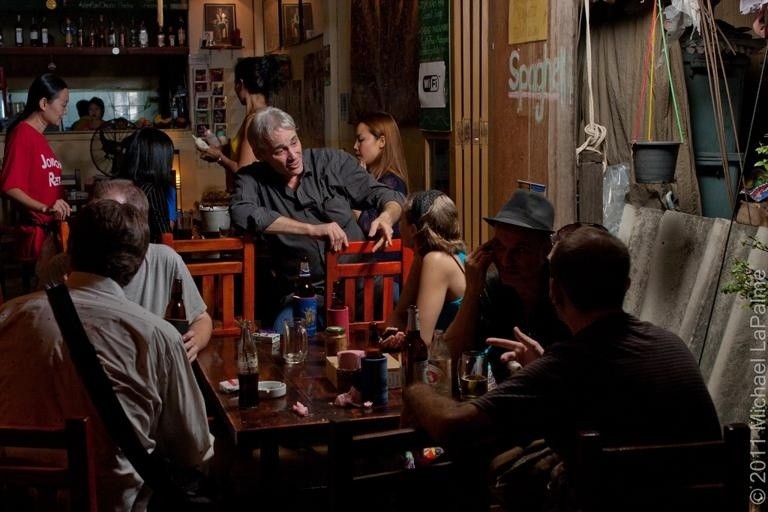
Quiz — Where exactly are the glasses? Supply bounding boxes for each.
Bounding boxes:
[557,220,609,238]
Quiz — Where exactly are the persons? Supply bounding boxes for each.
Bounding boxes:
[0,53,725,512]
[215,7,229,38]
[202,32,211,48]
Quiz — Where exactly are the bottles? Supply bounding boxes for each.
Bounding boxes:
[191,125,226,150]
[168,96,185,119]
[325,326,348,357]
[14,14,186,49]
[237,320,260,411]
[402,305,427,388]
[165,277,187,320]
[330,279,344,308]
[363,322,384,357]
[294,255,314,298]
[427,329,452,399]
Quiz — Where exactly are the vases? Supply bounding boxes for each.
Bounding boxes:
[630,140,682,183]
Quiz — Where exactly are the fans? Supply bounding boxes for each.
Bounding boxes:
[89,117,139,178]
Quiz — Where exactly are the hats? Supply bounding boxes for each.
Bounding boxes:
[482,190,556,234]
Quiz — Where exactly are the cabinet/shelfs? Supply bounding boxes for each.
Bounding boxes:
[0,0,191,217]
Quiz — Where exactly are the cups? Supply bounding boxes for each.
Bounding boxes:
[175,211,192,241]
[161,318,189,335]
[199,208,233,233]
[282,318,308,366]
[292,295,317,337]
[326,306,350,348]
[358,356,388,407]
[456,351,488,399]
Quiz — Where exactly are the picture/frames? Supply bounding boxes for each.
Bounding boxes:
[282,2,313,43]
[204,3,237,44]
[195,67,227,139]
